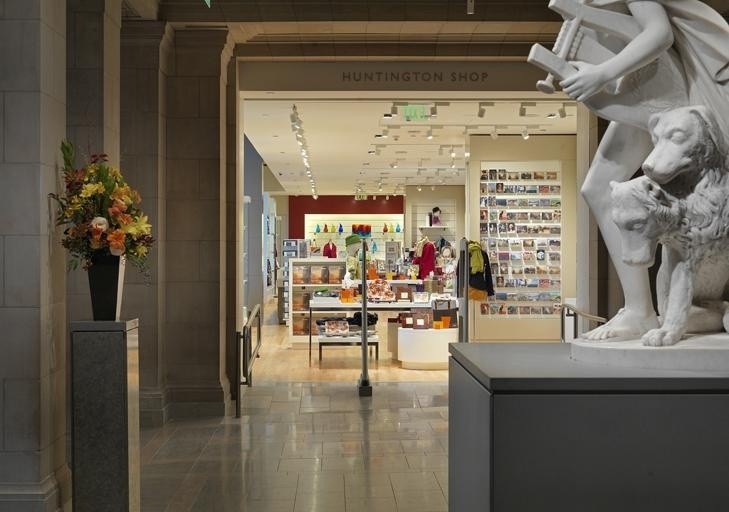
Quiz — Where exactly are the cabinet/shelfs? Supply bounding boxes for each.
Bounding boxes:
[310,232,403,259]
[478,159,563,317]
[288,260,346,343]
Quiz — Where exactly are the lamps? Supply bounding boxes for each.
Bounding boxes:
[290,105,319,201]
[357,101,573,200]
[467,0,475,14]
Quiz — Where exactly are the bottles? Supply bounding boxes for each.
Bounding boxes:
[425,212,433,227]
[312,239,316,246]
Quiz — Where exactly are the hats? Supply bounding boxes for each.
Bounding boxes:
[344,233,362,247]
[441,245,453,253]
[432,206,442,215]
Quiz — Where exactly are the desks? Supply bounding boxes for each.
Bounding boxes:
[318,330,379,361]
[387,319,460,368]
[309,295,459,368]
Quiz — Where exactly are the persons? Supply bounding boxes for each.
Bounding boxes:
[554,0,691,342]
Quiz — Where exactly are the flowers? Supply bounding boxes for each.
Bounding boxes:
[47,139,156,274]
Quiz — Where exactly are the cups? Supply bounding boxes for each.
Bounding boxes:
[432,316,451,329]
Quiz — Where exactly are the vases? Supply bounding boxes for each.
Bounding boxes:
[87,255,126,321]
